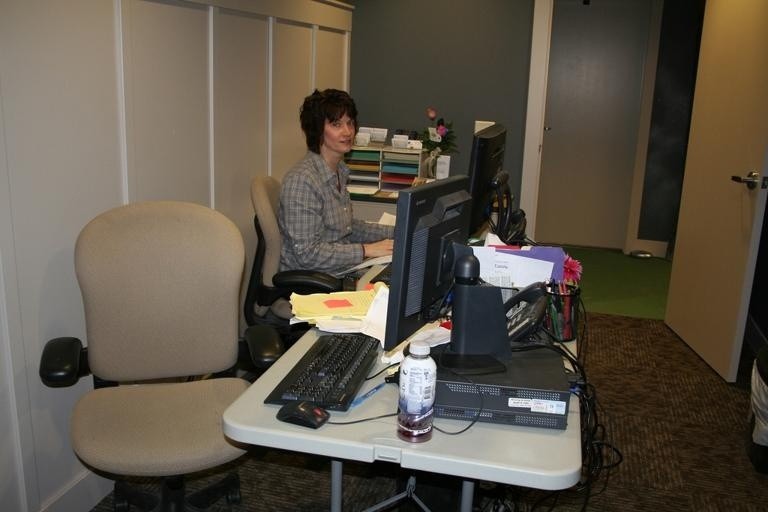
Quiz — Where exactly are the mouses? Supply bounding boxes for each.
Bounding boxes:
[277,400,331,429]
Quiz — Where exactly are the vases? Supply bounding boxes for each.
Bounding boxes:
[436,154,451,180]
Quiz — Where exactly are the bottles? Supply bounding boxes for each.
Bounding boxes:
[396,340,438,443]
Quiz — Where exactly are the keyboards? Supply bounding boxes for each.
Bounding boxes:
[264,333,380,411]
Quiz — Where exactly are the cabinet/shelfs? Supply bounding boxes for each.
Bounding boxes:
[343,144,428,190]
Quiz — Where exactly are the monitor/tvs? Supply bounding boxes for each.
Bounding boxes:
[470,123,506,233]
[383,175,512,373]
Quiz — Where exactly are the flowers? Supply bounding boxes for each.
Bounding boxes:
[425,107,460,155]
[564,254,581,285]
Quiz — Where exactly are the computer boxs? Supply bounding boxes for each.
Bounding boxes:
[433,341,571,429]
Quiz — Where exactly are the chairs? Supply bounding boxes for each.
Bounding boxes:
[40,201,284,512]
[244,176,342,335]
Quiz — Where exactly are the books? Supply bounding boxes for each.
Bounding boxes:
[291,281,387,337]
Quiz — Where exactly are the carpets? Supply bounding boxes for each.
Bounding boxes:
[87,309,768,512]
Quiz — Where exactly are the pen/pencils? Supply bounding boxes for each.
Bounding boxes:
[350,381,386,407]
[546,278,580,342]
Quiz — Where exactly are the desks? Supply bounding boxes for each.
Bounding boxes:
[222,264,582,512]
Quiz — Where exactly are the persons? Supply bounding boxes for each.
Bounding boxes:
[262,86,393,340]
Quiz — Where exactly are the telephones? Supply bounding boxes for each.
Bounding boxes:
[503,282,549,339]
